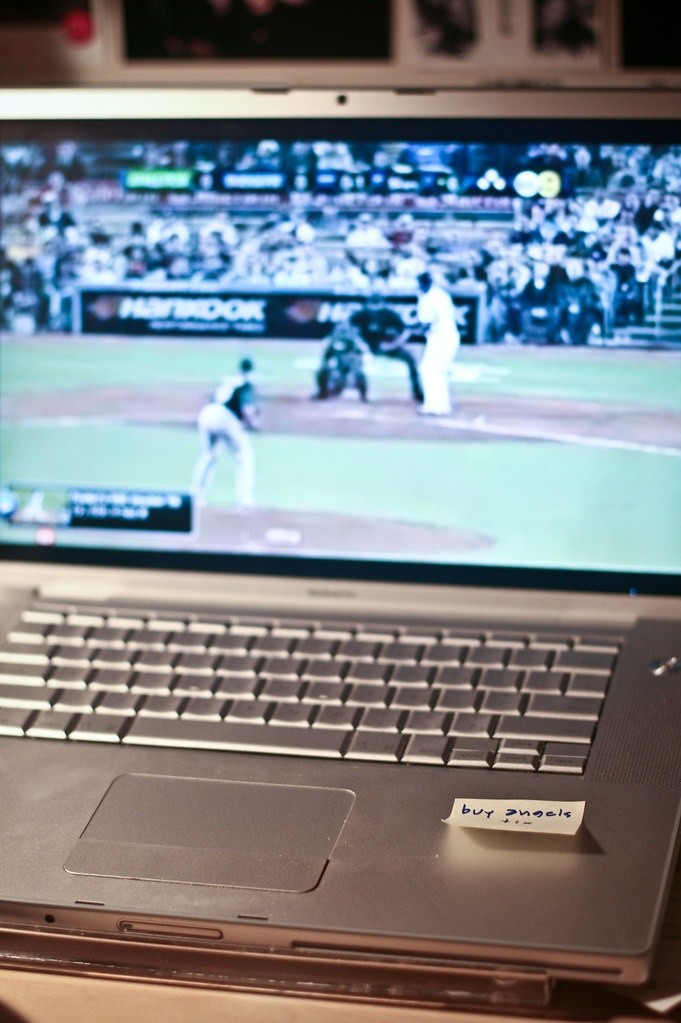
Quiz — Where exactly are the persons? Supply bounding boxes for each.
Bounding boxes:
[2,142,680,513]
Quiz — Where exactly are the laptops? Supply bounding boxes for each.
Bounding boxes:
[0,88,681,1017]
